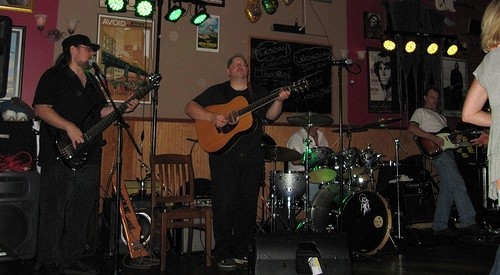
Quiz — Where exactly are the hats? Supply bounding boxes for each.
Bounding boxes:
[62,34,100,52]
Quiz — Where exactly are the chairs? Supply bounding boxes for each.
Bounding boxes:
[149,154,213,271]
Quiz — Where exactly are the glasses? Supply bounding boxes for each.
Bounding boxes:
[78,45,93,53]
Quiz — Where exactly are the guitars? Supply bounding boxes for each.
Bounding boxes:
[193,75,316,158]
[38,70,163,183]
[415,127,486,159]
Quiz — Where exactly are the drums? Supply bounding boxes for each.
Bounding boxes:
[337,146,366,180]
[303,146,339,185]
[307,184,394,257]
[361,148,383,176]
[267,170,309,211]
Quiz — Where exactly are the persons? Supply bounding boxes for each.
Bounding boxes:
[460,0,500,275]
[451,63,463,105]
[32,34,139,275]
[186,55,290,268]
[408,86,480,235]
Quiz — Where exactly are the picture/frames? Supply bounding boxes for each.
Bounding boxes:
[366,46,401,114]
[194,15,221,53]
[0,25,27,103]
[0,0,33,14]
[439,56,470,118]
[363,11,383,40]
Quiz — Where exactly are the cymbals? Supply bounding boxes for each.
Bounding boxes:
[364,115,410,128]
[261,131,276,153]
[260,147,302,161]
[331,123,370,133]
[286,114,335,126]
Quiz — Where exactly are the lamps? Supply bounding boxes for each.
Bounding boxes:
[340,49,369,75]
[34,12,81,43]
[104,0,129,14]
[133,0,156,18]
[382,33,459,57]
[163,0,186,24]
[188,4,210,27]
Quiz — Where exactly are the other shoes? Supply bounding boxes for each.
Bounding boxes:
[459,226,485,234]
[233,256,249,263]
[434,228,460,236]
[64,260,99,274]
[212,255,236,268]
